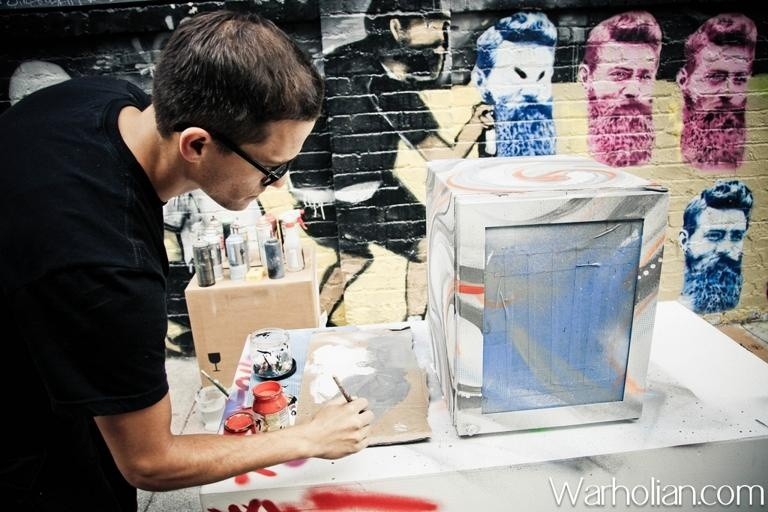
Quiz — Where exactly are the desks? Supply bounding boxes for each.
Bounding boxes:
[199,299,768,512]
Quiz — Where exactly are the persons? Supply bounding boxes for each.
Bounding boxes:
[675,15,756,169]
[468,10,558,156]
[0,8,374,510]
[678,178,756,313]
[578,12,662,166]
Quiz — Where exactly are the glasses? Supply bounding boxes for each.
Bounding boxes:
[173,115,290,190]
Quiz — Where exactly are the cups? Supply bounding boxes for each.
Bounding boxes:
[251,327,291,376]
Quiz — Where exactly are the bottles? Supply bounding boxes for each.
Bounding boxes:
[253,381,290,434]
[192,215,284,288]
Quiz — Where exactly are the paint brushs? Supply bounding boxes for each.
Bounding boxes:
[201,370,229,397]
[332,375,352,402]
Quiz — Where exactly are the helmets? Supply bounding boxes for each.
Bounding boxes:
[359,0,454,18]
